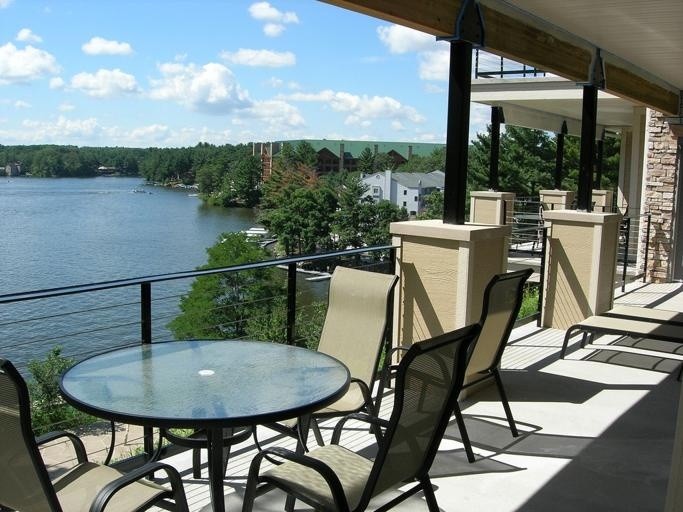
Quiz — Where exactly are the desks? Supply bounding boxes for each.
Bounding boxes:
[60,337,351,512]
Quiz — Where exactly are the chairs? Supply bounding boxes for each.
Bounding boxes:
[240,322,481,512]
[250,265,400,512]
[0,356,190,511]
[369,267,534,464]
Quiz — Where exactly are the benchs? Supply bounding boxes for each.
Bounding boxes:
[560,304,683,380]
